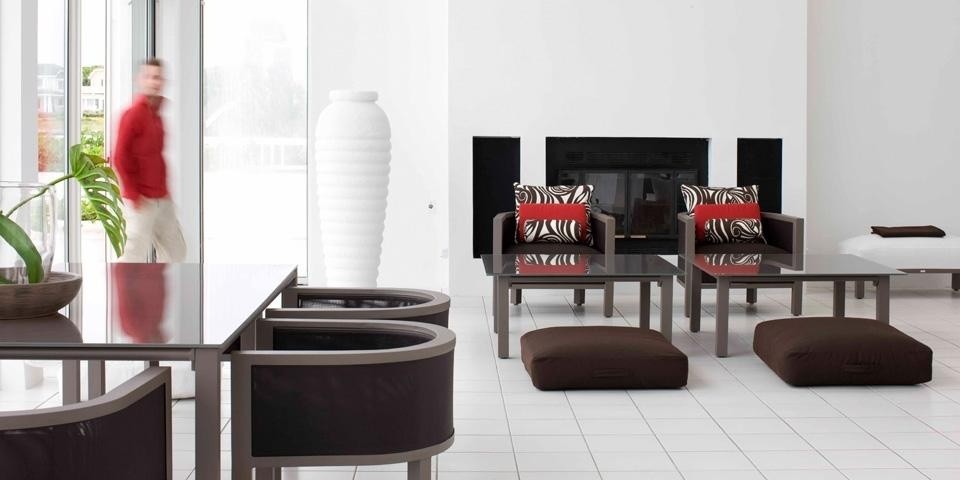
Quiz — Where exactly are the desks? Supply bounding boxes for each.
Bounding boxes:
[0,253,296,479]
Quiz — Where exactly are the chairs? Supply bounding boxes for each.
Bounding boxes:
[1,364,173,478]
[231,317,456,479]
[677,211,804,317]
[492,211,615,316]
[264,286,451,329]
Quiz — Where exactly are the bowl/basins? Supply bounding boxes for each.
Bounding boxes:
[0,268,83,321]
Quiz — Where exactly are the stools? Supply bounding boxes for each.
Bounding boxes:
[752,317,933,387]
[840,232,960,299]
[518,327,688,389]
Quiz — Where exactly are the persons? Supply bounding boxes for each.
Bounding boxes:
[113,58,188,264]
[111,265,168,344]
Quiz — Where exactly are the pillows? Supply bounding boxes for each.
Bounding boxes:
[518,201,586,241]
[694,204,761,245]
[695,252,762,274]
[680,183,760,219]
[522,219,582,245]
[515,253,591,278]
[704,218,766,245]
[513,181,596,247]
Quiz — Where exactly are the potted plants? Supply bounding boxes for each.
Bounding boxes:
[0,137,129,323]
